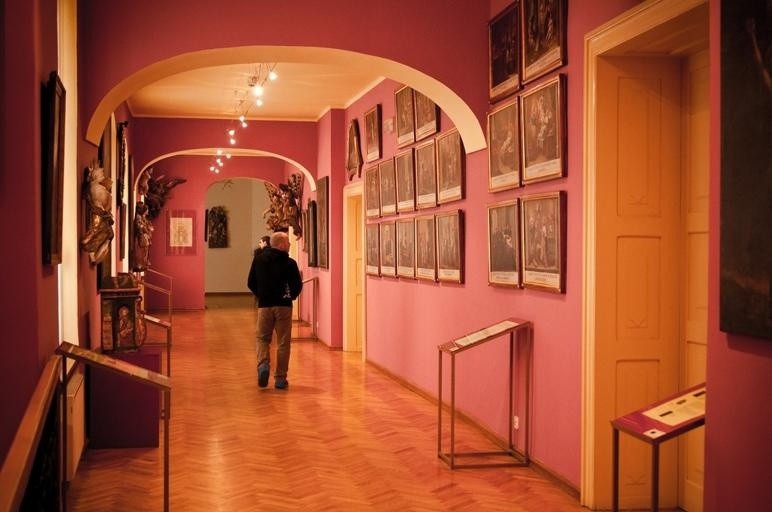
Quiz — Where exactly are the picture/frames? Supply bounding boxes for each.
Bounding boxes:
[44,69,67,267]
[486,94,520,194]
[300,175,330,270]
[360,82,468,287]
[520,190,568,294]
[519,72,568,186]
[522,0,568,85]
[485,198,520,287]
[487,0,521,104]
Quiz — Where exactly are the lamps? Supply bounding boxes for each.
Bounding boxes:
[204,63,279,175]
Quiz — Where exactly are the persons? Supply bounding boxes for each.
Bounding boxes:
[135,202,153,266]
[254,236,270,256]
[278,183,302,240]
[82,156,113,265]
[248,232,303,389]
[118,306,134,345]
[263,191,289,233]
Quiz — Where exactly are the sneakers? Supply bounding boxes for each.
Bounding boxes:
[257,365,269,386]
[275,380,287,388]
